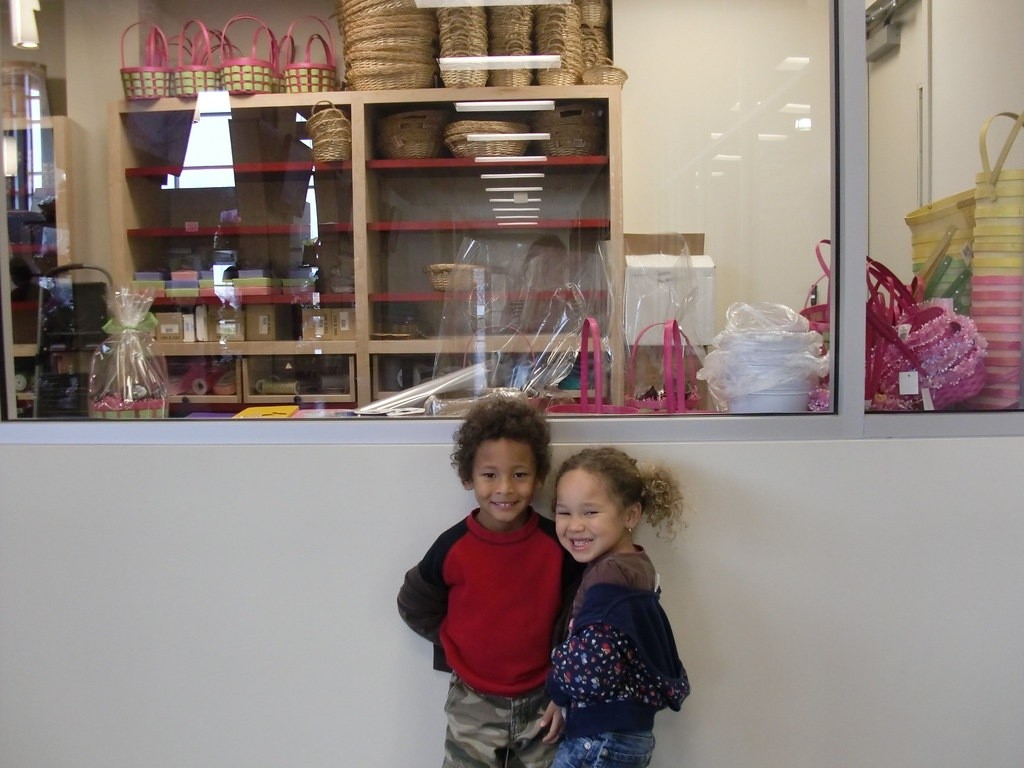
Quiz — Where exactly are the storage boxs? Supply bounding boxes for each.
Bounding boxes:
[622,233,716,348]
[148,302,298,343]
[301,306,357,343]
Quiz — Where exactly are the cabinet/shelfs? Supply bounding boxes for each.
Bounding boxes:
[0,116,74,422]
[106,84,625,412]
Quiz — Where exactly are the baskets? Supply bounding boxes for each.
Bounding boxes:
[88,333,170,421]
[119,0,629,158]
[422,263,491,291]
[797,239,991,411]
[382,322,423,339]
[462,317,700,414]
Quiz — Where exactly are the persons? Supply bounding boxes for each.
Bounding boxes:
[396,392,579,767]
[540,447,691,767]
